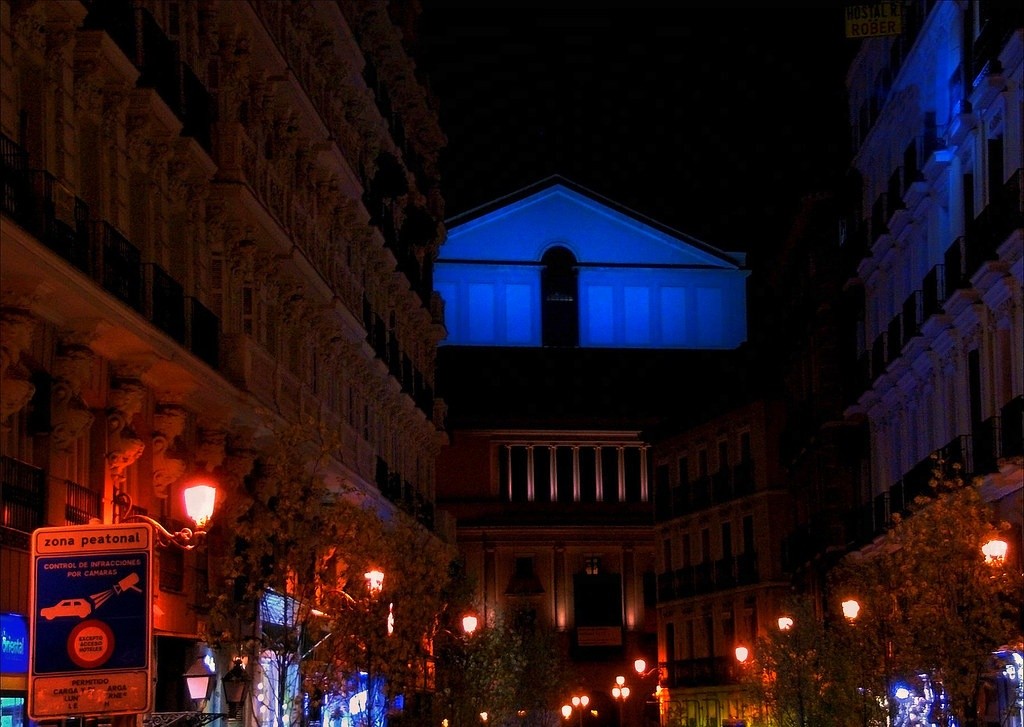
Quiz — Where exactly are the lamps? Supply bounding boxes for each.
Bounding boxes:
[112,470,226,557]
[634,656,667,688]
[840,597,865,630]
[982,540,1018,586]
[735,643,774,671]
[196,654,262,726]
[430,612,483,643]
[323,568,385,608]
[151,650,218,727]
[777,613,794,633]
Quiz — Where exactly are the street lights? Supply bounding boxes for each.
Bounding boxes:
[611,675,630,727]
[571,695,590,727]
[364,567,385,727]
[635,660,668,727]
[777,616,805,727]
[842,600,869,727]
